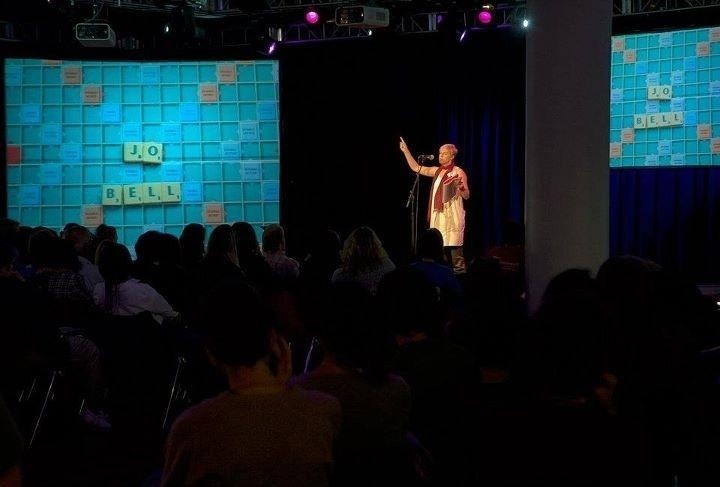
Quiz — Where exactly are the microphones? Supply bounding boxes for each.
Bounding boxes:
[419,155,435,161]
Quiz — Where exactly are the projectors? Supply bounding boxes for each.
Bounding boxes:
[335,6,389,29]
[76,23,116,48]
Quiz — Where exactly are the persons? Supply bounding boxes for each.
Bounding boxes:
[0,217,720,487]
[397,135,471,273]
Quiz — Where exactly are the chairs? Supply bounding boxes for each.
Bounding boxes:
[0,314,197,449]
